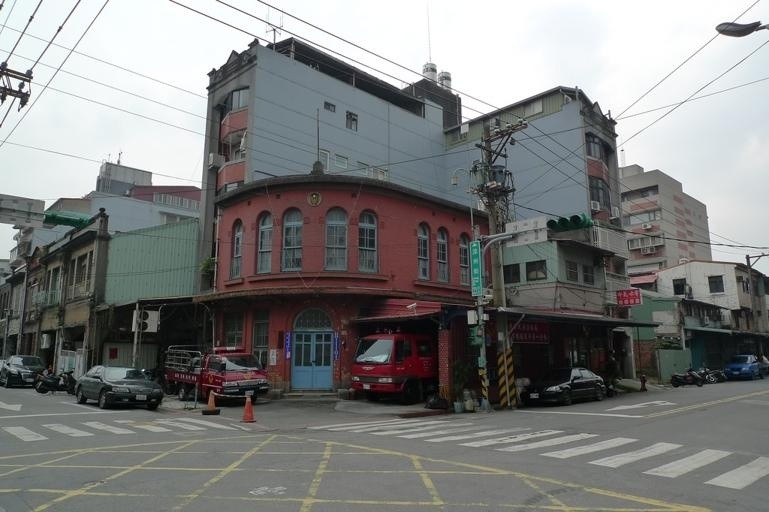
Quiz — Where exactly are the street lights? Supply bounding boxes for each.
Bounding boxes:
[716,21,769,37]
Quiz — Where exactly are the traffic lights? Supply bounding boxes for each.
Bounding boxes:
[547,212,591,234]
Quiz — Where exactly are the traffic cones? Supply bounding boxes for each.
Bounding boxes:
[239,394,257,423]
[200,388,222,415]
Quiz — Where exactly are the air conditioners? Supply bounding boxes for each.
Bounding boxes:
[641,224,651,229]
[648,247,655,254]
[591,201,600,210]
[612,206,619,217]
[641,248,648,254]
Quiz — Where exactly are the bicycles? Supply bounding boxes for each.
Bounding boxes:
[704,367,728,385]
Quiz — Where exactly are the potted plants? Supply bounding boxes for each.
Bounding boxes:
[453,362,469,412]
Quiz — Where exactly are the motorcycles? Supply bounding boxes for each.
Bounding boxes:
[670,361,704,388]
[35,366,79,394]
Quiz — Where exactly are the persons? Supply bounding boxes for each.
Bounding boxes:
[752,353,769,379]
[43,365,52,378]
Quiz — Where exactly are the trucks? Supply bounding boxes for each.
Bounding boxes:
[163,340,271,405]
[349,329,439,401]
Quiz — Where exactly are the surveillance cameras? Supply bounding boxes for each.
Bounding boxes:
[406,302,417,310]
[483,295,493,299]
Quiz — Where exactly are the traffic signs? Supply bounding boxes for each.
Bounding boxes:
[470,240,482,297]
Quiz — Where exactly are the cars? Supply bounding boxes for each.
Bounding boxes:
[73,365,166,412]
[723,354,761,382]
[0,353,47,389]
[524,366,607,407]
